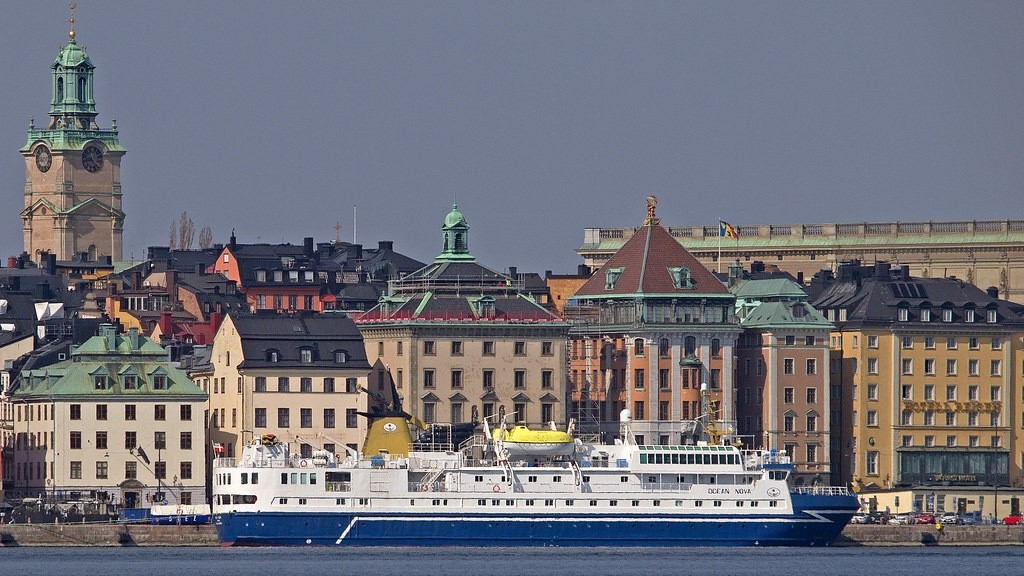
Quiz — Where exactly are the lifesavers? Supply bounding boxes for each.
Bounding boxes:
[492,485,500,493]
[300,460,307,467]
[176,509,183,515]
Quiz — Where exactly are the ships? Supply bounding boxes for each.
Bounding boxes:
[211,363,862,549]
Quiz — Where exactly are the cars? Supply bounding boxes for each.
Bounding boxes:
[851,508,994,525]
[1002,512,1024,525]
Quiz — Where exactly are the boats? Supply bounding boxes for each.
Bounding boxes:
[490,425,574,457]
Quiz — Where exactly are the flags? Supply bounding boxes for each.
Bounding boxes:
[720,220,739,239]
[138,446,150,465]
[212,441,224,453]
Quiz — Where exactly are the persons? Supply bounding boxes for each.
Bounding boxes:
[505,278,511,287]
